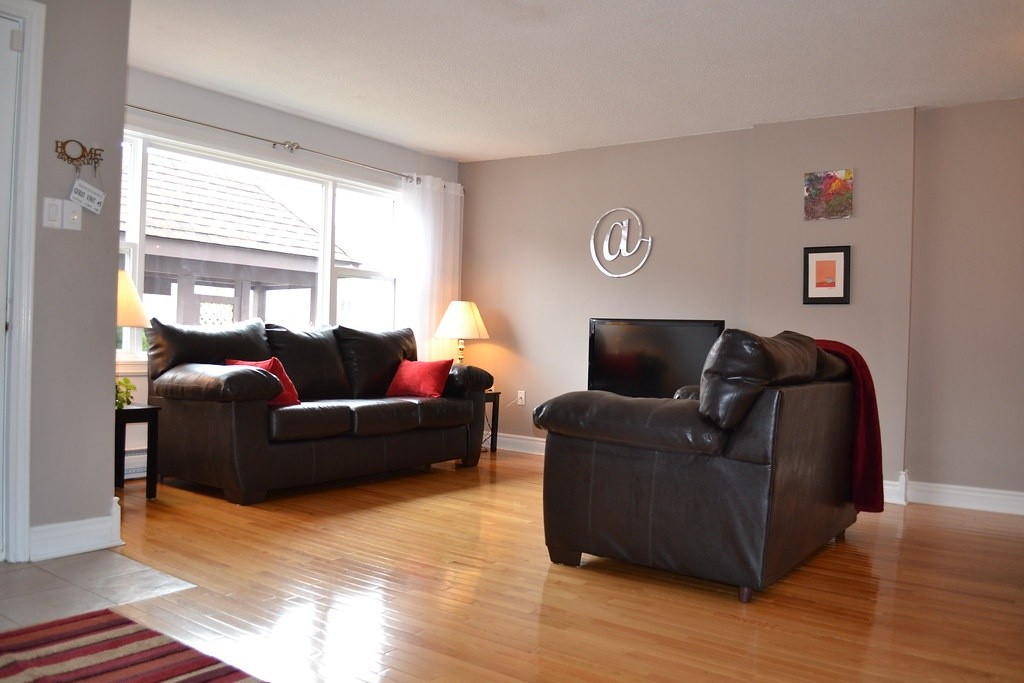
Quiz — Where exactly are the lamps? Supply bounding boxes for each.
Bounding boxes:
[116,269,149,330]
[434,301,489,364]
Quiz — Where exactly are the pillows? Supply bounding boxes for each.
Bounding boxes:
[385,359,454,398]
[225,357,302,405]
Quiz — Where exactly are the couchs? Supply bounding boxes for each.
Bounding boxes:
[533,330,857,602]
[145,319,494,504]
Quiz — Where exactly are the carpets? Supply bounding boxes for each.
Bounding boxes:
[0,607,264,683]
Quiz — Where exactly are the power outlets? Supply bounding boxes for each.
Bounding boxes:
[518,390,526,406]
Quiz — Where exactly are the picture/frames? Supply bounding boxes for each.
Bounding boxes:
[802,245,851,305]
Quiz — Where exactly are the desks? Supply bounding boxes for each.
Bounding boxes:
[115,403,162,500]
[485,391,501,452]
